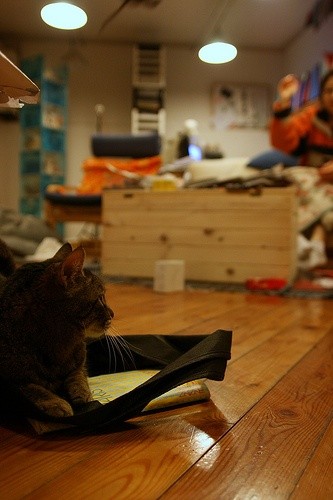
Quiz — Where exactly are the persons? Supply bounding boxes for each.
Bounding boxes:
[270,70,333,178]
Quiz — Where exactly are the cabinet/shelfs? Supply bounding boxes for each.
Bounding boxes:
[20,53,68,220]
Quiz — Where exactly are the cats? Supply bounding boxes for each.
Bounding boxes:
[0,241,140,437]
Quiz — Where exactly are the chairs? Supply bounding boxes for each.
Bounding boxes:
[45,135,163,227]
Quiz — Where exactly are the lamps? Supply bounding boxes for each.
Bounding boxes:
[197,33,238,64]
[41,0,89,31]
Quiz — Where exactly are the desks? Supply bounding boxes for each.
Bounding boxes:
[101,189,301,288]
